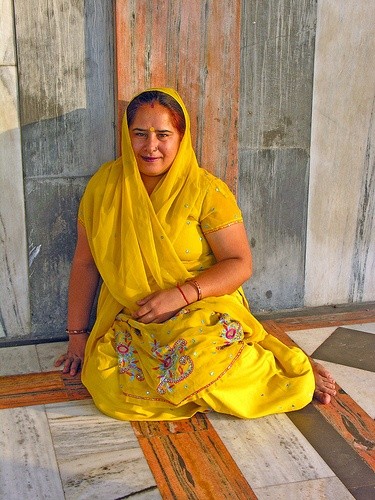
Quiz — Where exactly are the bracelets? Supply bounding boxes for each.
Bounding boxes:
[65,329,87,336]
[183,279,203,301]
[176,284,191,307]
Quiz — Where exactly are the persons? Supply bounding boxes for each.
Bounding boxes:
[54,88,336,422]
